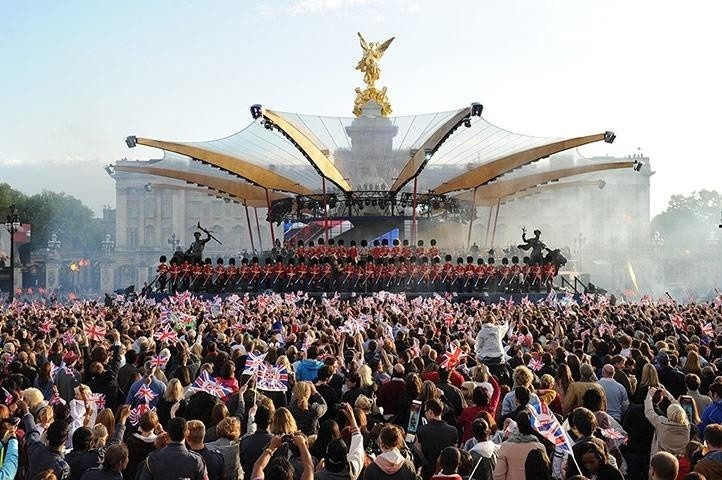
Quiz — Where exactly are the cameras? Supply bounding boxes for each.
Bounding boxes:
[282,435,294,443]
[336,402,347,411]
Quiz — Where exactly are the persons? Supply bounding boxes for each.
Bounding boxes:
[0,282,721,480]
[363,42,378,86]
[522,230,552,265]
[156,240,556,294]
[185,232,210,264]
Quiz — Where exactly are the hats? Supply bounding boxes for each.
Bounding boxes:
[323,439,350,474]
[215,334,227,342]
[272,322,281,330]
[63,350,80,365]
[291,325,298,332]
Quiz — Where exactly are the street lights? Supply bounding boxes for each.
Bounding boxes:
[4,205,20,304]
